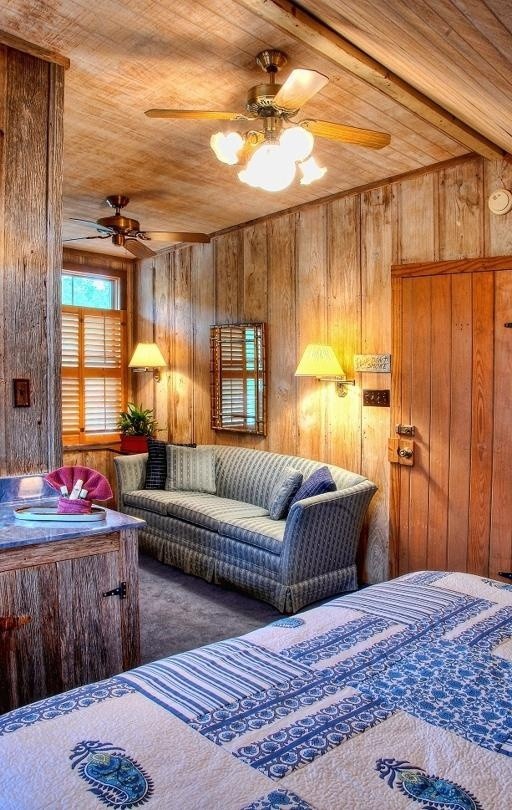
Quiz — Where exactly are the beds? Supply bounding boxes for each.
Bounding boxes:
[0,570,512,810]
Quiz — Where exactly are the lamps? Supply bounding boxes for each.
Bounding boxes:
[295,343,356,398]
[210,119,327,193]
[128,343,167,383]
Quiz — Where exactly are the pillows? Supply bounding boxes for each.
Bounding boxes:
[268,466,336,521]
[143,440,217,494]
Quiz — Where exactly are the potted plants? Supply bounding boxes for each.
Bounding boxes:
[115,401,158,453]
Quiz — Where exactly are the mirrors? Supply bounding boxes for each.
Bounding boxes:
[210,324,267,435]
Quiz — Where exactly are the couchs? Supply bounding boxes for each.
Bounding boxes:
[114,444,379,615]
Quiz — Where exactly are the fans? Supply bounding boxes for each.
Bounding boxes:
[143,51,393,193]
[61,195,212,259]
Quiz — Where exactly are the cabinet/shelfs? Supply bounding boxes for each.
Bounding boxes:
[0,33,70,478]
[0,530,139,716]
